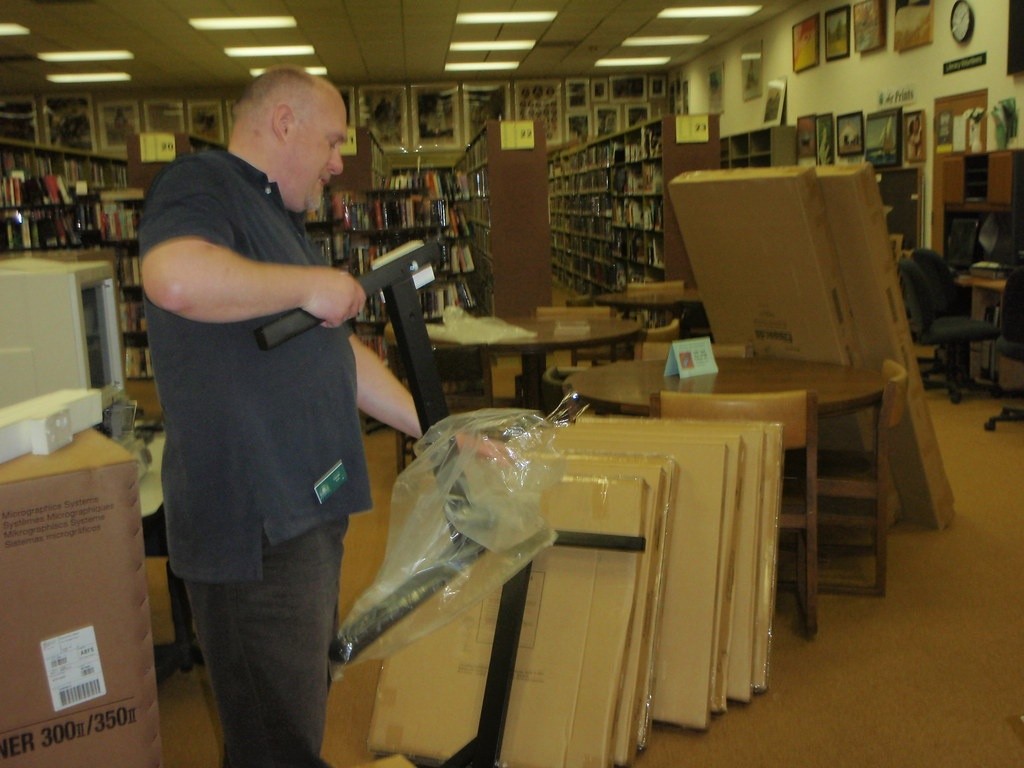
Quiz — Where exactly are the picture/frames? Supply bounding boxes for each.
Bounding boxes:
[0,86,356,156]
[815,113,835,165]
[791,12,818,70]
[866,108,902,168]
[707,62,725,116]
[837,112,864,156]
[796,115,815,158]
[894,0,934,52]
[359,85,409,154]
[825,4,851,60]
[743,39,764,100]
[904,109,927,163]
[463,82,511,145]
[411,83,461,152]
[514,80,562,145]
[762,76,786,127]
[853,0,886,52]
[565,76,693,141]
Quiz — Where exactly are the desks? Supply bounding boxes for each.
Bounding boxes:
[953,269,1006,383]
[563,355,884,486]
[384,320,642,474]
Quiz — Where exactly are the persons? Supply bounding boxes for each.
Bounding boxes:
[140,66,516,768]
[907,115,923,159]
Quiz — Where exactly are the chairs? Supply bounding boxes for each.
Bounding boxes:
[984,274,1024,430]
[813,357,908,600]
[520,304,746,361]
[898,245,996,404]
[648,390,820,639]
[537,366,566,416]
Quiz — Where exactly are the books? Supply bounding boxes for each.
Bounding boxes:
[545,125,667,297]
[305,159,492,373]
[0,146,154,381]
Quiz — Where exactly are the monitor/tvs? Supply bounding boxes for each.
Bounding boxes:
[947,217,978,267]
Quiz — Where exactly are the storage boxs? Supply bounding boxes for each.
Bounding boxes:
[0,427,163,768]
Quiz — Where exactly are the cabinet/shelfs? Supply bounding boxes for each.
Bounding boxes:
[100,189,441,384]
[721,128,796,167]
[453,122,550,317]
[0,138,126,252]
[550,114,720,321]
[125,131,227,193]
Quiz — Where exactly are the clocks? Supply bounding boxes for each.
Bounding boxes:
[951,0,974,43]
